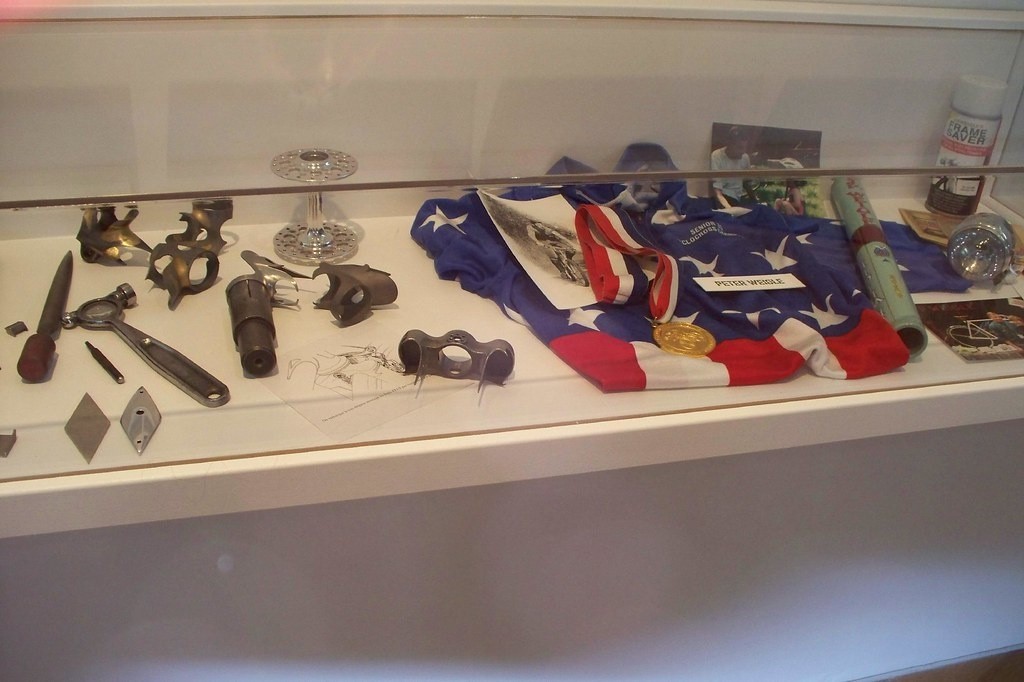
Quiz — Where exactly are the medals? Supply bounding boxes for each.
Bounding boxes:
[653,322,716,357]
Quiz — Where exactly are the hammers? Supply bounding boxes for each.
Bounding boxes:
[59,283,231,408]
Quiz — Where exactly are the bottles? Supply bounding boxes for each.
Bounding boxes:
[924,76,1010,216]
[948,213,1014,281]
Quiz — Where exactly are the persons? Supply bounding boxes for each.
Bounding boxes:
[987,312,1024,354]
[711,126,804,215]
[527,219,577,280]
